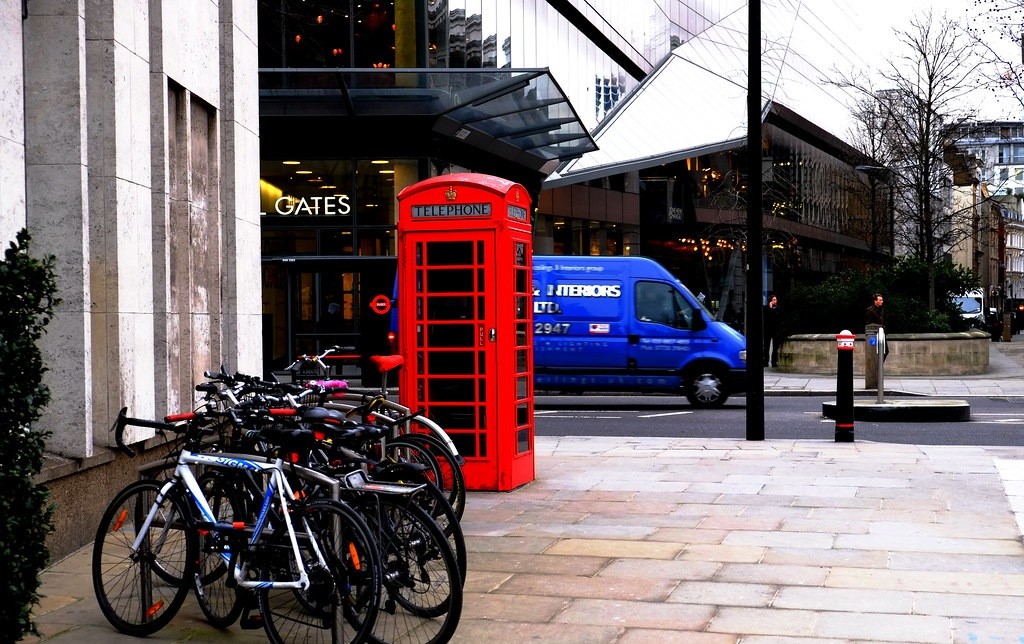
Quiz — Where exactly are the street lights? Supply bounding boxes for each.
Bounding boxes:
[855,166,896,272]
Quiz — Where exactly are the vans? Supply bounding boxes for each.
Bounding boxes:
[952,296,985,328]
[387,254,746,410]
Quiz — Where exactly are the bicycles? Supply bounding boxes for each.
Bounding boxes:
[94,345,467,644]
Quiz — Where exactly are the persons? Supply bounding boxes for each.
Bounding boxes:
[866,293,889,362]
[639,285,687,323]
[764,295,787,368]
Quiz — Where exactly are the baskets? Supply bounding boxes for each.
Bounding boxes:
[240,391,264,409]
[275,394,321,430]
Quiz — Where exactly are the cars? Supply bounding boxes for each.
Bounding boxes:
[985,307,1001,328]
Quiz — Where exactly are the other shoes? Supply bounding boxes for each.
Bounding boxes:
[764,362,769,367]
[773,363,778,367]
